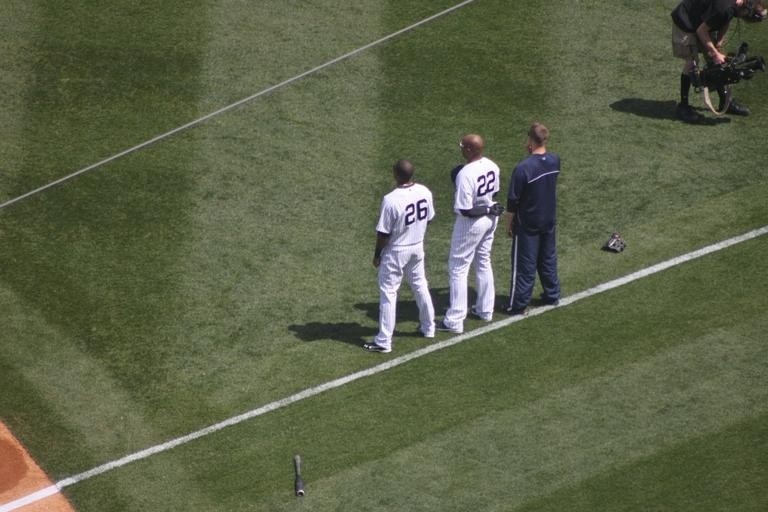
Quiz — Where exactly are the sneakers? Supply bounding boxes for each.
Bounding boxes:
[500,305,524,314]
[434,320,464,334]
[677,103,699,123]
[360,343,392,353]
[417,325,434,338]
[467,308,482,320]
[719,99,751,116]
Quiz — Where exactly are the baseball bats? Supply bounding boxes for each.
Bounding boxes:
[294,454,304,497]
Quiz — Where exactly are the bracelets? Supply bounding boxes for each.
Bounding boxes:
[375,253,379,257]
[487,207,490,215]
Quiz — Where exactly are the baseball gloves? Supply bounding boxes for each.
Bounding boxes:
[603,233,625,253]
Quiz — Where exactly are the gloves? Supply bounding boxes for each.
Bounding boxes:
[488,203,505,215]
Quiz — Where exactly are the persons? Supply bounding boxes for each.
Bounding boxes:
[494,123,560,315]
[670,0,768,123]
[433,134,505,332]
[360,158,436,355]
